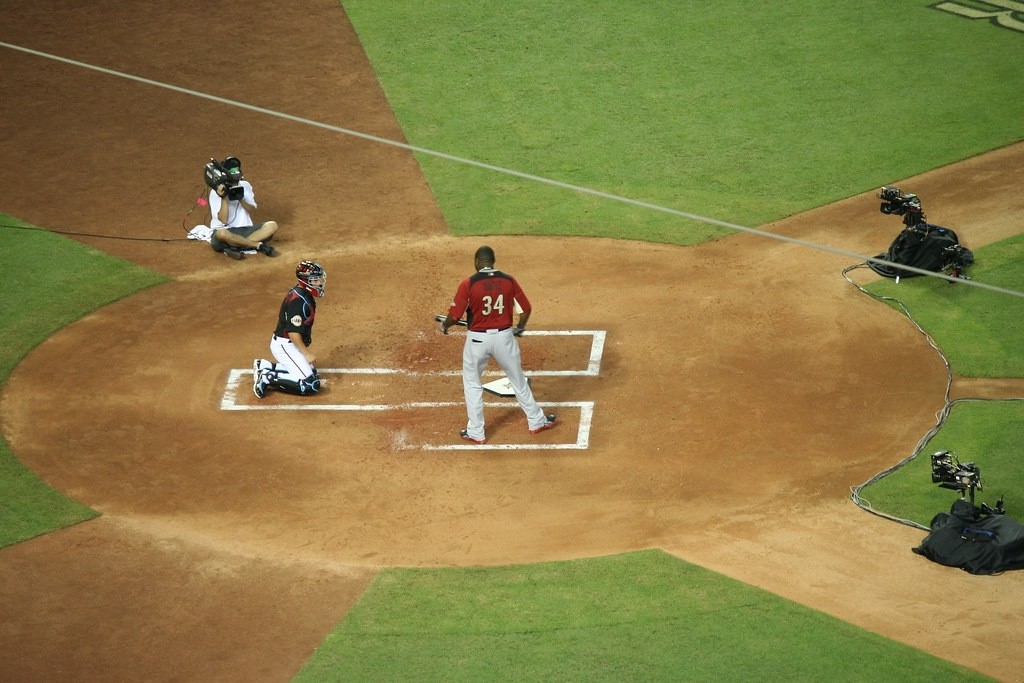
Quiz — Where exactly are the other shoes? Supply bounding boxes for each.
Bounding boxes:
[259,242,275,256]
[253,357,272,399]
[223,246,245,260]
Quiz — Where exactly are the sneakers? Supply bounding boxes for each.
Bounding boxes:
[529,414,557,434]
[459,429,487,445]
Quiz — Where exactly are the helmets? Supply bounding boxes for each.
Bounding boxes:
[295,260,324,286]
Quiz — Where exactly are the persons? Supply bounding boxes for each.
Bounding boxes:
[439,245,556,444]
[209,156,279,259]
[252,260,327,398]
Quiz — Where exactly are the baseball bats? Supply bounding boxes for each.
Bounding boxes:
[435,314,521,337]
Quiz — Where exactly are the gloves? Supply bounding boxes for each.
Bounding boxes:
[439,321,452,334]
[512,326,526,337]
